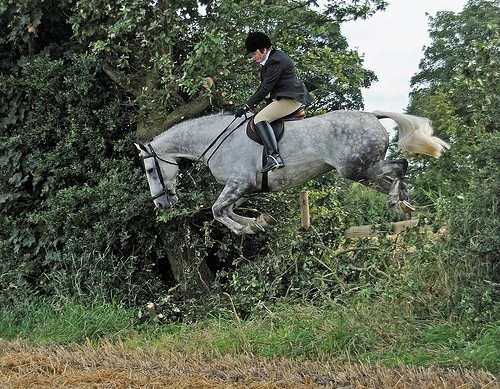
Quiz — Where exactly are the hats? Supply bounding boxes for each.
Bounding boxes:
[243,32,272,56]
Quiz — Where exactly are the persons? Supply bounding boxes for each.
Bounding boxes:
[235,32,307,173]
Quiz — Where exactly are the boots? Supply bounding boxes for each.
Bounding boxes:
[254,120,285,173]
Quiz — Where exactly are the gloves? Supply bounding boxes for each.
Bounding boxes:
[234,108,246,118]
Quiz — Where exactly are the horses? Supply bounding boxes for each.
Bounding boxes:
[133,110,451,235]
